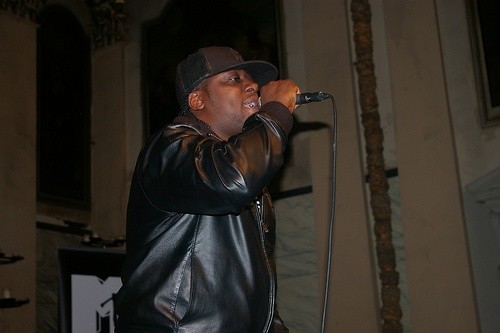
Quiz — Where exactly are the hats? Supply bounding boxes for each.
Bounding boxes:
[175,46,279,114]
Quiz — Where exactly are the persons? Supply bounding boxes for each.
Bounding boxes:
[115,46,301,333]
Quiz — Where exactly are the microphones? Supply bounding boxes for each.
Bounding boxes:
[258,91,332,109]
